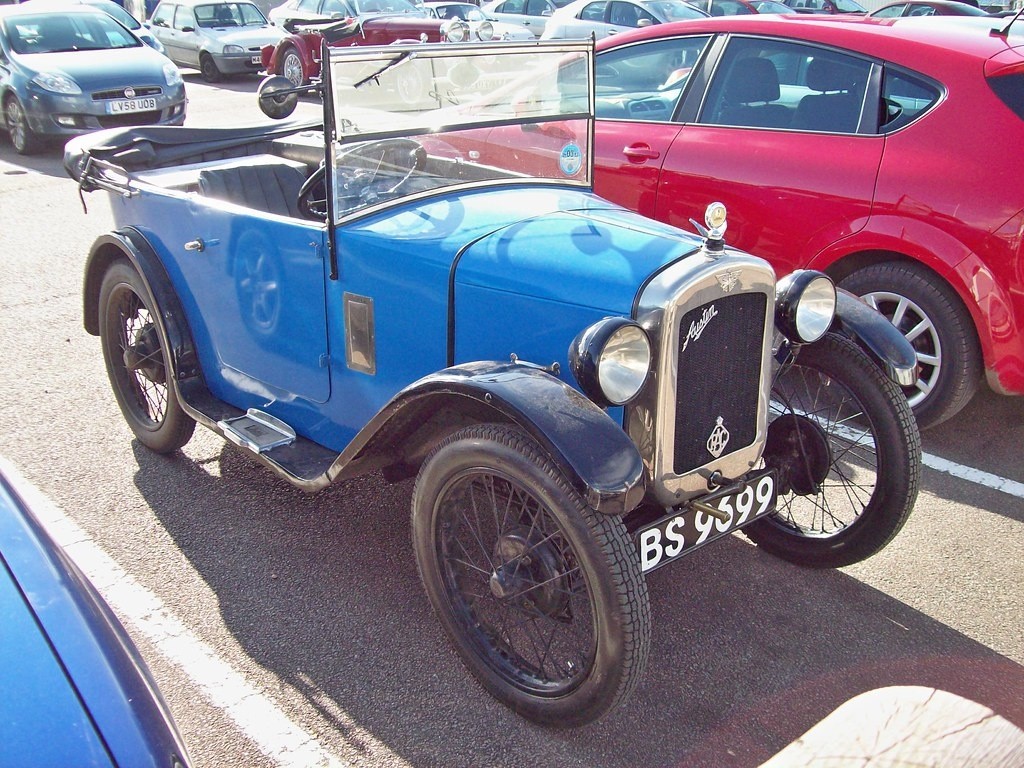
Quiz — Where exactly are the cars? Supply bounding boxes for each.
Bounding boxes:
[408,9,1024,436]
[149,1,295,84]
[60,31,924,731]
[0,0,190,156]
[259,0,1024,112]
